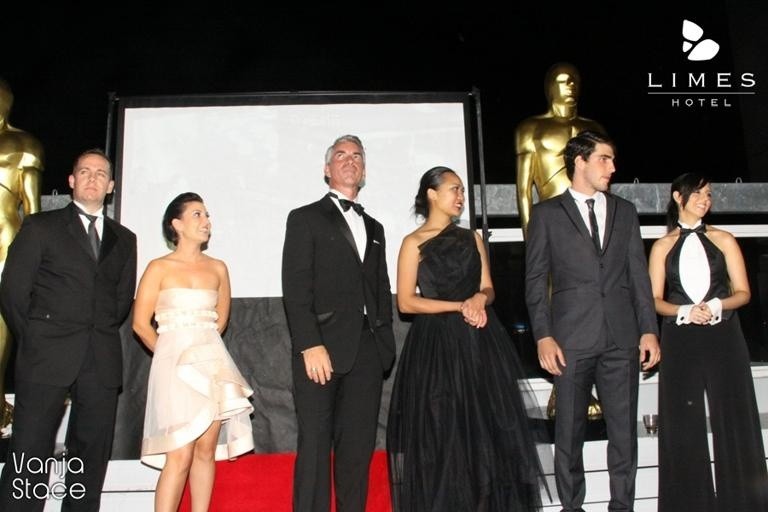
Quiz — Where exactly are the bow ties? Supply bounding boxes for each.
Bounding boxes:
[339,199,364,215]
[681,224,707,238]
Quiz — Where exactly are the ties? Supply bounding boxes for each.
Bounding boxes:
[585,199,600,253]
[85,215,100,259]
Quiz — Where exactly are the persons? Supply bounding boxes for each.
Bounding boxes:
[132,192,257,509]
[526,131,663,511]
[514,64,612,422]
[389,168,542,512]
[0,148,136,512]
[649,165,766,512]
[0,81,45,431]
[283,135,394,511]
